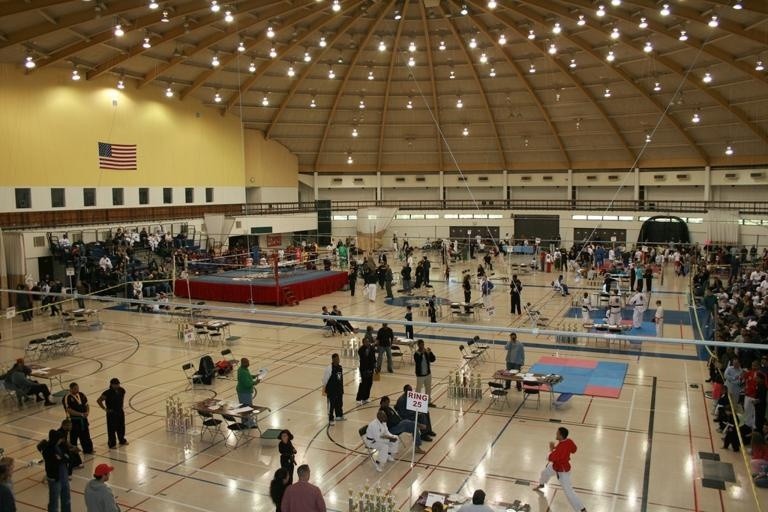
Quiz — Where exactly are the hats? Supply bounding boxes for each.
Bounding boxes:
[277,430,292,440]
[94,464,113,477]
[110,378,119,384]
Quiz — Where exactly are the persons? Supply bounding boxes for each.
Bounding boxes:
[505,332,525,391]
[269,467,290,511]
[434,232,569,303]
[457,489,495,512]
[321,323,437,471]
[221,233,430,302]
[404,306,413,340]
[510,275,522,315]
[236,358,262,428]
[531,426,588,512]
[1,467,14,511]
[43,432,71,512]
[14,226,220,322]
[5,358,129,453]
[279,465,327,512]
[569,242,667,338]
[429,295,436,323]
[666,238,768,488]
[1,458,15,496]
[322,305,359,335]
[278,430,297,485]
[431,501,443,512]
[85,463,120,512]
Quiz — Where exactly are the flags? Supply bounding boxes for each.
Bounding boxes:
[98,141,138,171]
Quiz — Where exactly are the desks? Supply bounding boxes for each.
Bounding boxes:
[410,491,530,512]
